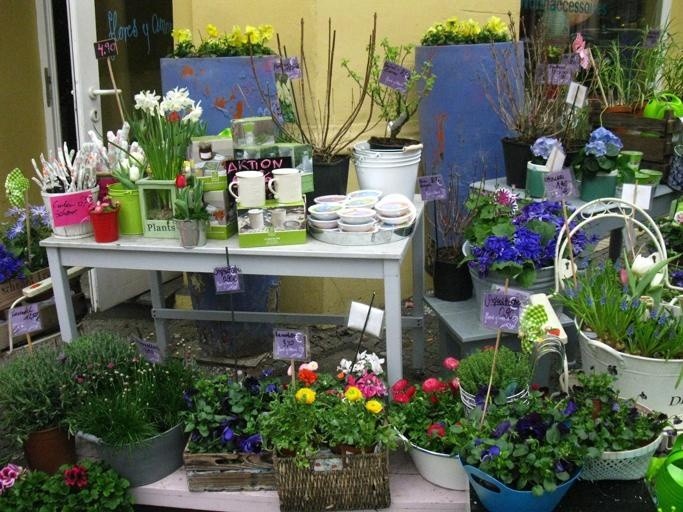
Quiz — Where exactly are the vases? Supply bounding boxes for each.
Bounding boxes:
[108,182,143,234]
[97,421,187,487]
[277,447,293,457]
[582,166,617,201]
[648,248,683,270]
[573,315,683,433]
[0,268,49,311]
[365,446,376,452]
[173,218,207,248]
[526,162,549,198]
[90,209,119,243]
[563,139,589,168]
[574,398,663,480]
[25,289,53,303]
[134,177,181,239]
[340,444,358,456]
[415,42,524,248]
[160,55,282,358]
[667,145,683,192]
[328,442,341,452]
[458,451,584,512]
[96,171,118,203]
[462,240,563,321]
[41,186,99,241]
[184,432,276,492]
[393,426,470,490]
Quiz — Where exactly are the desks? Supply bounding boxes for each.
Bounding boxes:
[469,176,674,264]
[40,200,426,408]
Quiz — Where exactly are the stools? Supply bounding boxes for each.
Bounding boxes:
[423,295,575,368]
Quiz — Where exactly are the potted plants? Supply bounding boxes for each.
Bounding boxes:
[455,347,532,419]
[477,10,579,189]
[420,145,499,302]
[1,343,89,464]
[631,10,683,119]
[546,45,563,64]
[341,39,435,207]
[585,23,634,135]
[236,12,384,214]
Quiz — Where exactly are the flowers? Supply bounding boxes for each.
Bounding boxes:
[530,138,564,165]
[86,194,119,214]
[456,185,601,289]
[31,141,98,192]
[387,344,500,456]
[548,246,683,388]
[109,142,149,189]
[91,85,208,212]
[171,174,214,221]
[458,382,585,496]
[555,32,595,148]
[0,203,53,272]
[572,127,636,185]
[0,244,26,284]
[420,16,513,46]
[71,325,202,460]
[45,460,136,512]
[0,463,49,512]
[178,366,277,454]
[256,350,386,458]
[88,123,131,173]
[564,364,683,461]
[166,23,276,56]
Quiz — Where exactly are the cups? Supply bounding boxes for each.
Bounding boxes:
[267,209,286,225]
[244,209,263,228]
[228,171,266,208]
[267,168,303,204]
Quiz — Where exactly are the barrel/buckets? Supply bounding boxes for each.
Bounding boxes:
[351,141,423,203]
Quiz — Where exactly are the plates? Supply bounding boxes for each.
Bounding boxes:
[307,220,416,245]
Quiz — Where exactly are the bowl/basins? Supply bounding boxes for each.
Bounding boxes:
[376,202,412,224]
[308,195,345,232]
[337,190,383,232]
[284,221,299,230]
